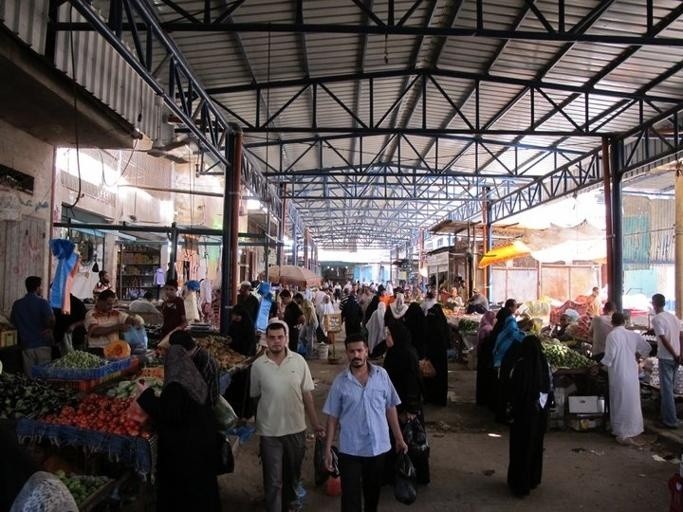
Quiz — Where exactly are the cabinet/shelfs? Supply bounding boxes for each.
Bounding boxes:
[445,311,482,362]
[116,241,161,300]
[544,368,590,432]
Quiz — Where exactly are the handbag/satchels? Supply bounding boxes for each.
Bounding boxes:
[212,433,236,476]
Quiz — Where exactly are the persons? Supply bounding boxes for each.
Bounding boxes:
[604,312,651,441]
[82,291,144,356]
[247,319,324,511]
[466,289,530,422]
[128,261,213,329]
[168,331,220,442]
[323,332,410,512]
[372,325,431,487]
[10,275,54,374]
[506,336,552,496]
[137,346,221,510]
[229,280,463,408]
[93,270,115,301]
[651,294,683,430]
[549,287,618,360]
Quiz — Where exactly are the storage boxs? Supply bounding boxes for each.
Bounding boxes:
[569,395,599,413]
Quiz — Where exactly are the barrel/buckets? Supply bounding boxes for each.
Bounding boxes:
[316,345,328,360]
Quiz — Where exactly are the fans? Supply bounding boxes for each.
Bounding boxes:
[450,219,498,258]
[108,96,194,164]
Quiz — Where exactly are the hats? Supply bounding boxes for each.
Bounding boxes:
[473,288,480,293]
